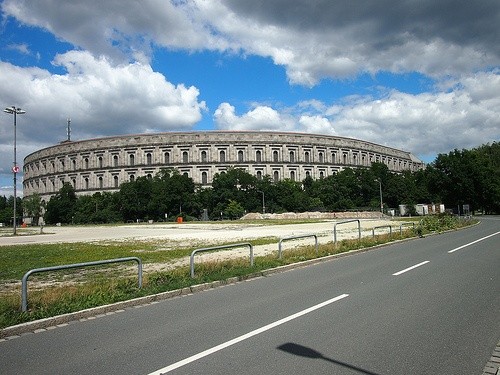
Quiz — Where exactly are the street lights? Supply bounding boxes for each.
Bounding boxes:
[4,106,28,236]
[257,190,265,214]
[374,179,383,214]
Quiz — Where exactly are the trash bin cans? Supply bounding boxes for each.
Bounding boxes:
[177,217,183,224]
[23,223,26,227]
